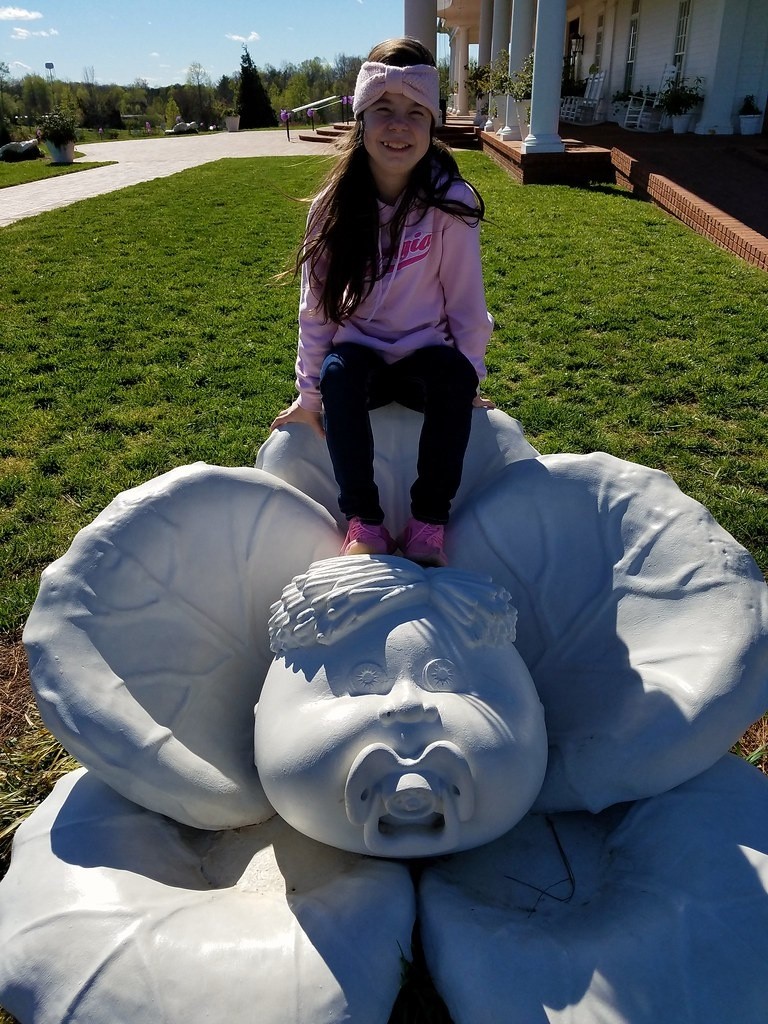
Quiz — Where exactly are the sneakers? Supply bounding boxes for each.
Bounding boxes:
[338,515,394,557]
[395,516,448,568]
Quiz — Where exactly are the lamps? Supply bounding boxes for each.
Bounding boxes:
[570,33,584,55]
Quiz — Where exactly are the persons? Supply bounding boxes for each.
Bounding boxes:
[268,39,495,568]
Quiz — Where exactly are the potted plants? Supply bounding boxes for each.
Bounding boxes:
[660,75,706,134]
[221,108,240,132]
[465,58,489,121]
[447,80,458,110]
[36,100,78,164]
[502,50,564,143]
[640,104,661,132]
[485,49,509,128]
[738,94,763,135]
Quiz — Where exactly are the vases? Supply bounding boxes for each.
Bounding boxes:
[613,101,641,127]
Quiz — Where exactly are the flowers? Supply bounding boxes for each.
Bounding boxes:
[37,129,42,137]
[612,89,654,116]
